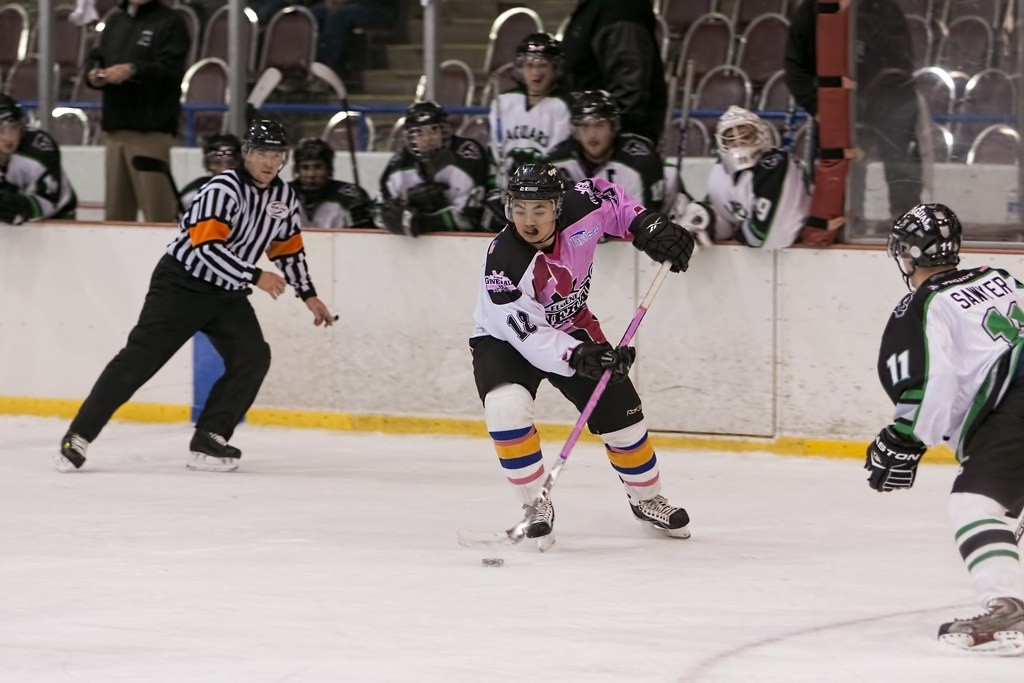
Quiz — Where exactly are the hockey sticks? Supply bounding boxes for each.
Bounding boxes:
[679,59,696,158]
[308,61,363,186]
[457,261,673,547]
[781,94,796,150]
[244,57,287,131]
[489,70,506,168]
[129,154,188,219]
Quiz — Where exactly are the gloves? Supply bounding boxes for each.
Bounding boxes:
[568,341,636,385]
[379,199,425,237]
[628,211,695,273]
[864,425,927,492]
[400,181,450,211]
[0,182,31,225]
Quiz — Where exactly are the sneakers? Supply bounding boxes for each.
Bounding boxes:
[619,474,692,539]
[934,598,1024,656]
[526,495,556,552]
[186,427,242,472]
[59,427,89,472]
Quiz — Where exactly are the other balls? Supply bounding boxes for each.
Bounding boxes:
[482,558,504,567]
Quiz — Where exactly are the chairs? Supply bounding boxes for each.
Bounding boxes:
[0,0,1024,256]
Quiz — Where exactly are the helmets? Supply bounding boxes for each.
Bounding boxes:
[0,94,26,133]
[402,101,452,160]
[243,120,290,170]
[202,134,242,174]
[887,203,961,267]
[504,159,566,223]
[512,31,565,79]
[568,89,621,141]
[714,106,774,173]
[293,139,333,196]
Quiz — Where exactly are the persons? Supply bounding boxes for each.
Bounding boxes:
[81,0,189,223]
[168,0,922,253]
[864,203,1024,657]
[56,118,335,472]
[469,159,695,553]
[0,94,77,226]
